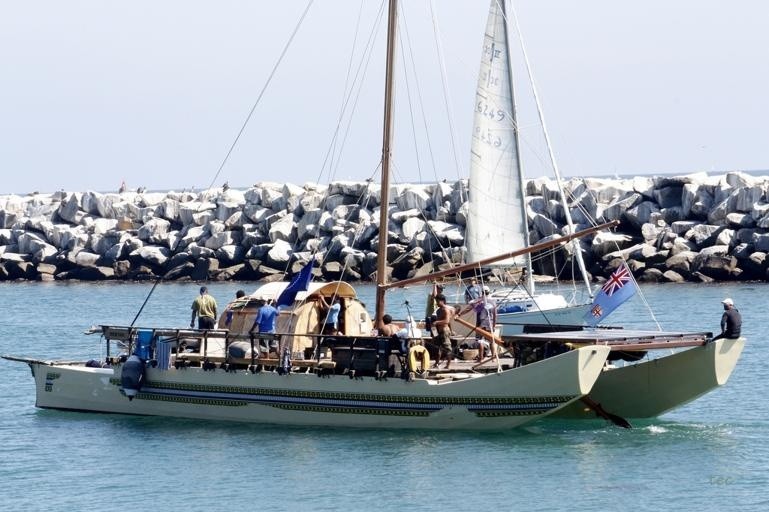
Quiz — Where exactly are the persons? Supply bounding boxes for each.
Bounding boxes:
[710,298,744,343]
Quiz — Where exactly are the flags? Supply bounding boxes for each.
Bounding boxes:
[270,255,316,306]
[582,259,641,332]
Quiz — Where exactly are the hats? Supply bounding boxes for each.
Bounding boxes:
[721,298,733,306]
[433,294,445,300]
[481,286,490,293]
